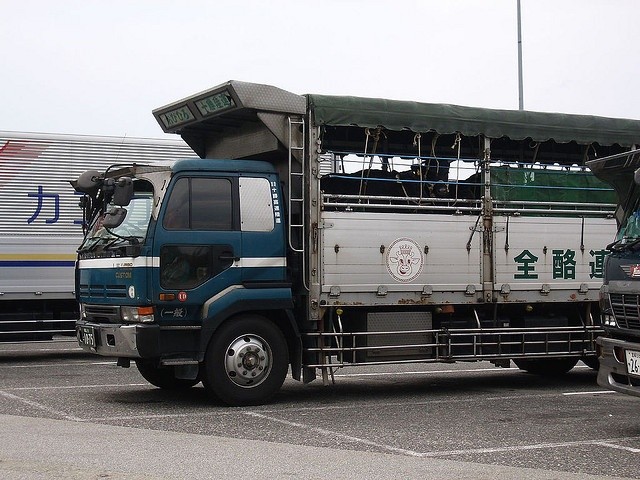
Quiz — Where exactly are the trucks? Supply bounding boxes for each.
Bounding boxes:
[585,137,640,399]
[72,80,640,405]
[0,129,205,345]
